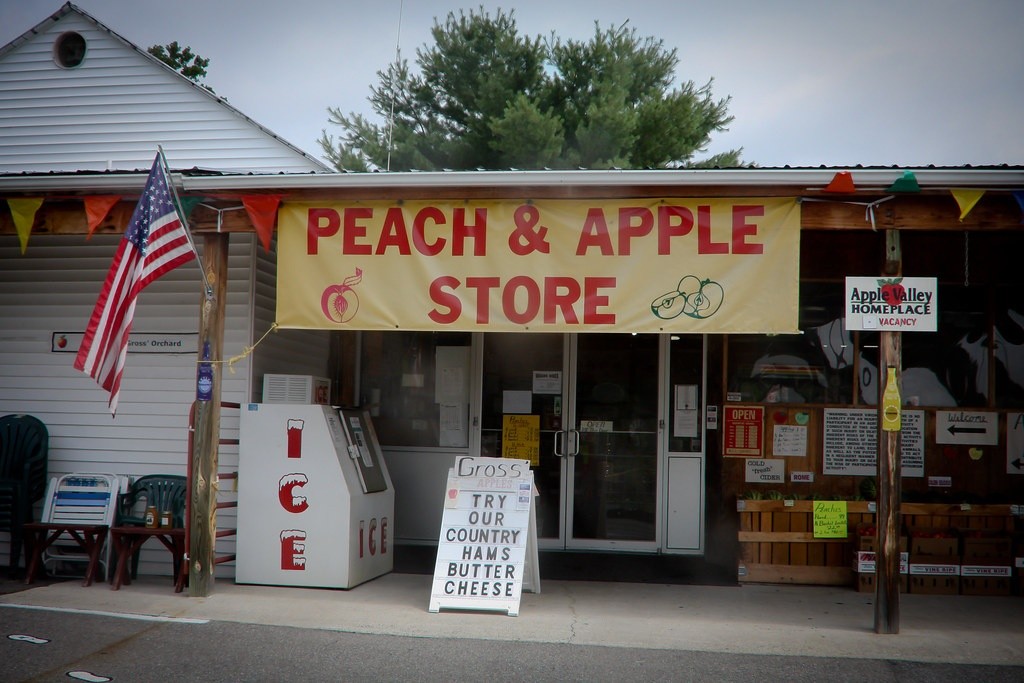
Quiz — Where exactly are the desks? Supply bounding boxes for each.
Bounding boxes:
[111,526,186,593]
[22,523,109,587]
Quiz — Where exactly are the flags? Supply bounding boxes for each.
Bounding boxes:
[71,147,198,418]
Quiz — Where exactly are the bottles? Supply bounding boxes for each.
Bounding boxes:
[197,342,213,400]
[883,365,901,431]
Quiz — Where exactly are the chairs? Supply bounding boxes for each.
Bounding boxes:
[0,413,188,589]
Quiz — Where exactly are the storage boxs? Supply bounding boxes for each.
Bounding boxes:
[857,522,1013,596]
